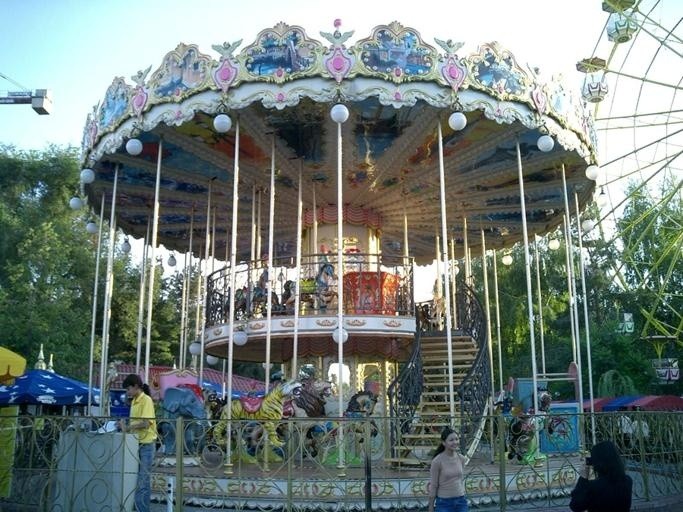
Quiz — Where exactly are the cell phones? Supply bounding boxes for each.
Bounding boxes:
[585,456,592,466]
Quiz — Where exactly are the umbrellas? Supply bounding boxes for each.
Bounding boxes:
[0,368,112,416]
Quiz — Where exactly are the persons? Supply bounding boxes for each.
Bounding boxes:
[115,374,158,512]
[618,415,649,449]
[429,429,469,512]
[569,442,633,512]
